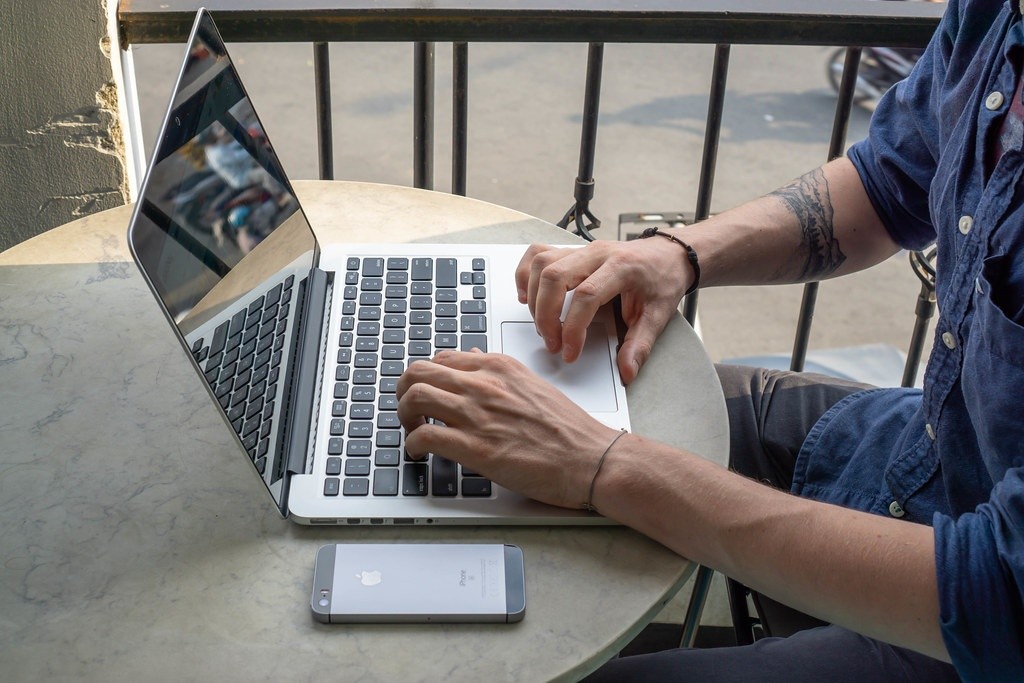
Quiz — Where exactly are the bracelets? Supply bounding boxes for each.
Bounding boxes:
[639,228,702,295]
[583,429,627,513]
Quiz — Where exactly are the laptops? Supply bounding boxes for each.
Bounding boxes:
[126,7,633,526]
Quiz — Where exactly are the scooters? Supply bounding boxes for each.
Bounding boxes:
[824,46,931,105]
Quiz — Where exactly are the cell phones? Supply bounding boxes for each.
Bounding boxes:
[310,544,527,623]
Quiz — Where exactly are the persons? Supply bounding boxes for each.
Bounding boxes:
[397,0,1023,683]
[194,124,292,208]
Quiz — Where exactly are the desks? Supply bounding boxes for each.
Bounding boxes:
[0,179,732,683]
